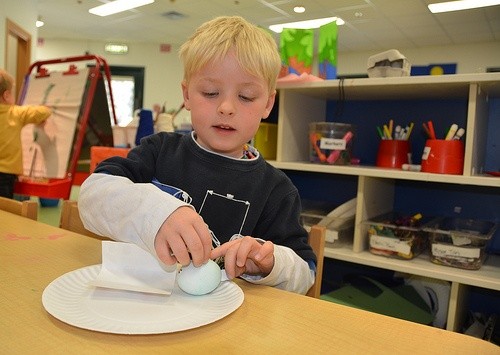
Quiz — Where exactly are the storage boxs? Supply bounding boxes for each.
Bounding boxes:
[423,217,495,270]
[364,210,426,260]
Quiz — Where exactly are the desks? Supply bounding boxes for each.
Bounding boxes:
[0,206,500,355]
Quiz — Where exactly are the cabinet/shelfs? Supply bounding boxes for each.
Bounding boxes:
[257,73,500,342]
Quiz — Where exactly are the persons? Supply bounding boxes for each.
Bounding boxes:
[0,67,50,199]
[77,16,317,296]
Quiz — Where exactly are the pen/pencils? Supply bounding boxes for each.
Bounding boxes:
[377,120,413,140]
[454,129,465,140]
[447,124,458,140]
[423,122,436,140]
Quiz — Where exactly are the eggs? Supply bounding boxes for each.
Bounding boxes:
[176,258,222,296]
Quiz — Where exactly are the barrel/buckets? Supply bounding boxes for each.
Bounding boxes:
[377,139,408,169]
[421,139,464,176]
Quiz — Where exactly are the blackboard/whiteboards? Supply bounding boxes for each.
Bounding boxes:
[14,52,118,180]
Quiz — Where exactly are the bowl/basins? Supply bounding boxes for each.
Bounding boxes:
[308,121,355,166]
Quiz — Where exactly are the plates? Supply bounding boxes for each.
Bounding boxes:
[42,264,245,336]
